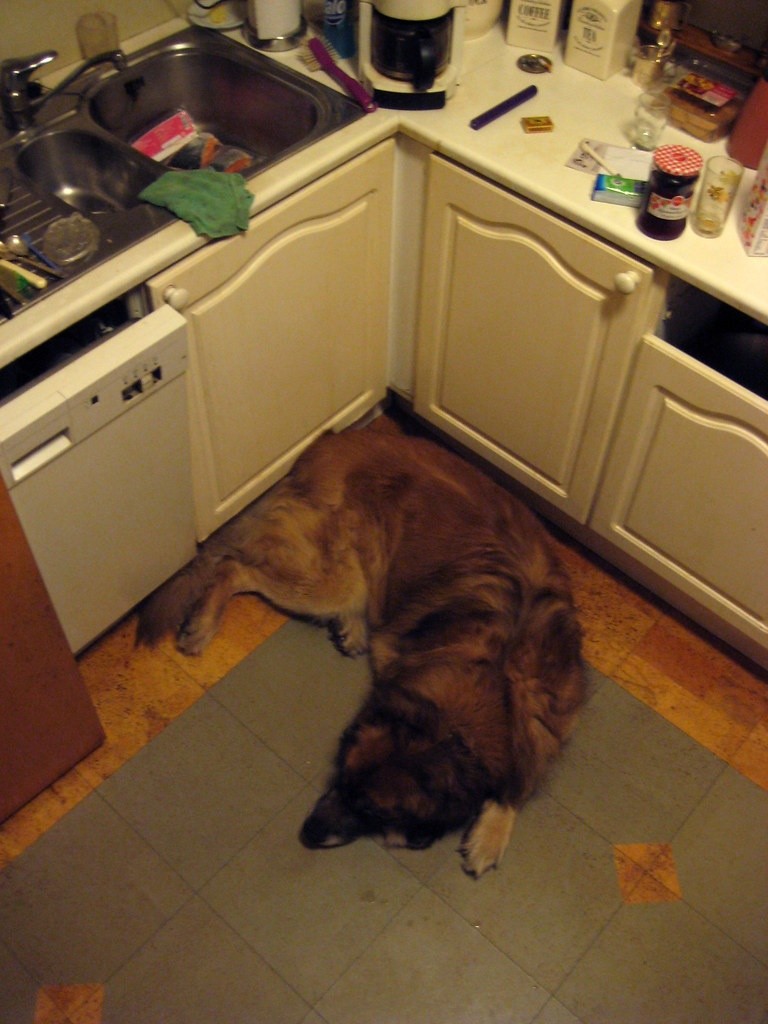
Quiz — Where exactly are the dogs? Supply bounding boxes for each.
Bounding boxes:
[133,429,586,880]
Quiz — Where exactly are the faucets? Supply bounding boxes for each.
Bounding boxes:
[0,49,129,131]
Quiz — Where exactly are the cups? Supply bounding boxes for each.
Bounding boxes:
[370,0,454,91]
[693,156,744,239]
[634,92,672,152]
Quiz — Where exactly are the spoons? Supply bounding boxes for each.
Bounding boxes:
[7,235,36,261]
[0,241,66,279]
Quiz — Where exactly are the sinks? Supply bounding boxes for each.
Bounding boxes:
[14,128,159,216]
[75,39,333,183]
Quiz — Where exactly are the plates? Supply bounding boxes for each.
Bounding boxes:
[187,0,245,30]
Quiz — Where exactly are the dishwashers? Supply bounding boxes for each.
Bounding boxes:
[0,295,197,658]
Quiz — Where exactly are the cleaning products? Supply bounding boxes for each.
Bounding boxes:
[323,0,348,57]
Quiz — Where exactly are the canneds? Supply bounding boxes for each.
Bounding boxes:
[636,145,703,242]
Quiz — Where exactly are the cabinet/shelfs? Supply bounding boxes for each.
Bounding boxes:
[144,130,395,544]
[411,139,766,667]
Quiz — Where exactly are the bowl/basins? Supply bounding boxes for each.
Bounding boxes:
[454,0,504,41]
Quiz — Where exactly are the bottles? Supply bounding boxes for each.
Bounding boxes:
[737,144,768,257]
[726,77,768,170]
[563,0,643,81]
[506,0,563,52]
